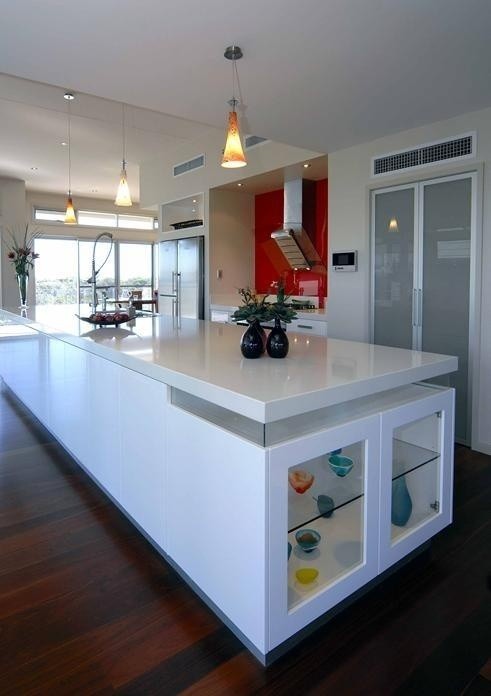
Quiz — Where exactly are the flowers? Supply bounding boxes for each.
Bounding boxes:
[0,220,46,310]
[263,278,298,324]
[244,288,265,323]
[229,287,265,322]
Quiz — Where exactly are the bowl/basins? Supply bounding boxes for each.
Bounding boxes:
[295,569,319,585]
[295,530,321,553]
[288,471,314,494]
[327,456,353,476]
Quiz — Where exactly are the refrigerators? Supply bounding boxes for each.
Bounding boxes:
[157,237,204,320]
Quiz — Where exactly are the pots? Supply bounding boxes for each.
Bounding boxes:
[291,299,311,304]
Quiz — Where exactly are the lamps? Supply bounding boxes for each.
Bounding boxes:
[63,91,77,224]
[113,103,133,207]
[220,45,248,169]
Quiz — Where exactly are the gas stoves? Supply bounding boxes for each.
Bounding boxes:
[254,301,321,314]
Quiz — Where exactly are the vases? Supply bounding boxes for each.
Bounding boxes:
[256,324,268,344]
[239,324,264,360]
[16,273,29,310]
[265,317,290,359]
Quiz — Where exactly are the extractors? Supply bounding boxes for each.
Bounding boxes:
[270,177,322,271]
